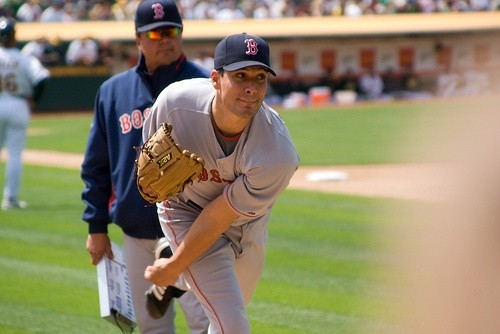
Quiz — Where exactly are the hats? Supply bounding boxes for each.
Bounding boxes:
[135,0,183,33]
[213,32,276,79]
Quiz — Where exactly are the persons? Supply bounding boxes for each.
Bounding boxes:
[137,32,299,334]
[177,0,499,102]
[0,0,140,71]
[0,15,49,211]
[80,0,209,334]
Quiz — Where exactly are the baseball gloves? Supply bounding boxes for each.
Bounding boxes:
[133,122,205,205]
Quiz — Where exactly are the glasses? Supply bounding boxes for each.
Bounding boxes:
[144,27,182,38]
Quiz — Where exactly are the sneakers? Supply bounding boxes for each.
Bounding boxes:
[146,237,188,319]
